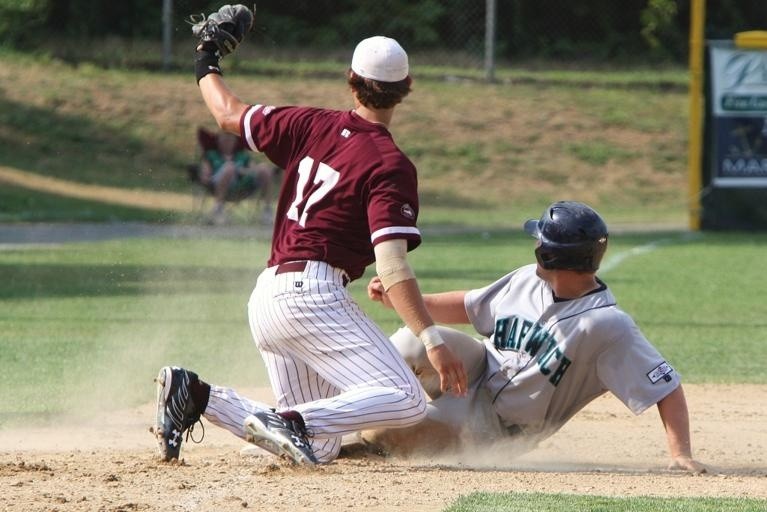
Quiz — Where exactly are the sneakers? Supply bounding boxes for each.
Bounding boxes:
[244,407,318,465]
[155,365,200,462]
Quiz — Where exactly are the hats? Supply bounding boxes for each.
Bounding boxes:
[351,35,409,83]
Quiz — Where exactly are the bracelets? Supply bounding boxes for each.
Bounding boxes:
[420,324,446,350]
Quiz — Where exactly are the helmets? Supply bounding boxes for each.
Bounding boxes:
[523,200,609,271]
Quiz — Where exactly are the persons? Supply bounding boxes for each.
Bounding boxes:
[200,130,276,225]
[340,202,706,476]
[158,3,467,467]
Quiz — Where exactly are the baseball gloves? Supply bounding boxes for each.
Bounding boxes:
[192,4,254,60]
